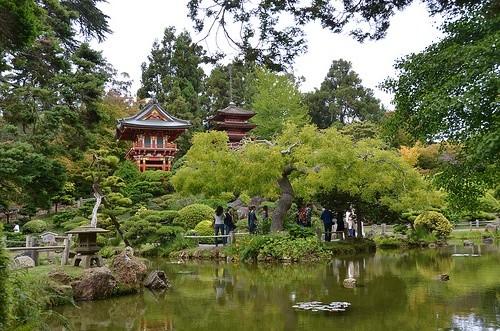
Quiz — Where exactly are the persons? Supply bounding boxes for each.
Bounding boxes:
[320,205,335,242]
[262,205,269,233]
[213,205,227,246]
[336,213,357,238]
[213,266,235,307]
[224,206,238,246]
[12,221,20,233]
[321,260,365,295]
[304,202,313,227]
[247,204,260,234]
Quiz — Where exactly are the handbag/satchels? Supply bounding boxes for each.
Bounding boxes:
[332,221,335,225]
[210,221,215,229]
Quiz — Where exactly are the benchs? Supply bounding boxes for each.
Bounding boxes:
[317,227,348,241]
[184,235,232,246]
